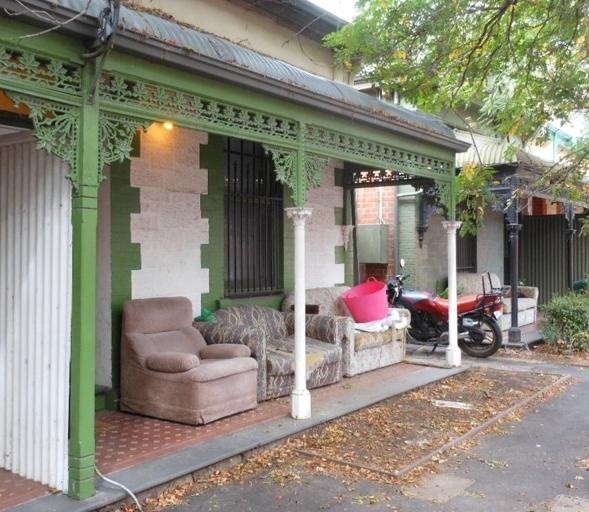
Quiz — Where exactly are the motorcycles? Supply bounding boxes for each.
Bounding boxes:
[388,259,510,358]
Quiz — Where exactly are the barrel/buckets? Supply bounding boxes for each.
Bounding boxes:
[341,282,386,322]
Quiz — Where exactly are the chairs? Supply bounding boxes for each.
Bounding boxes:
[479,271,539,332]
